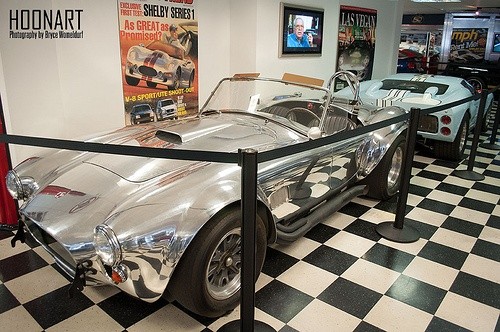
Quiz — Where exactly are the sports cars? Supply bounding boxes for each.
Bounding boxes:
[445,59,499,90]
[332,72,494,157]
[5,78,410,319]
[126,41,196,91]
[399,49,429,74]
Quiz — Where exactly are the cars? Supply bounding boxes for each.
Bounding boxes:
[156,98,178,120]
[340,38,371,82]
[130,104,155,125]
[452,49,481,65]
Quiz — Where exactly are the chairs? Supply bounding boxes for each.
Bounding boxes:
[307,114,358,136]
[260,106,296,123]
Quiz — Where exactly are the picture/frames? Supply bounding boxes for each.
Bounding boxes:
[278,2,325,58]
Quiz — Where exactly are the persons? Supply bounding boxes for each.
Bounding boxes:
[169,24,193,56]
[307,33,317,47]
[422,36,435,58]
[351,31,371,45]
[287,18,312,48]
